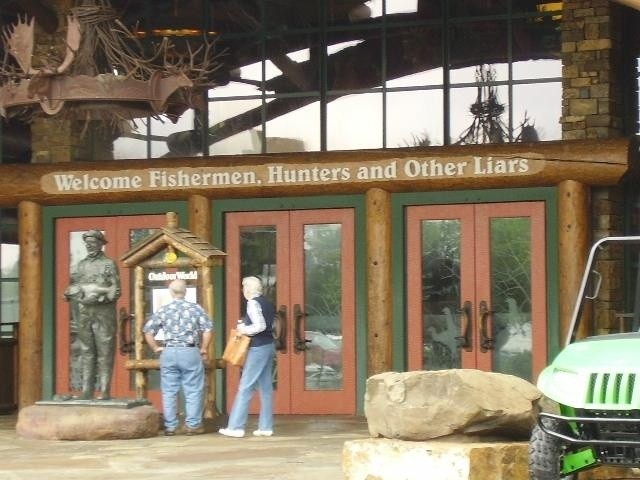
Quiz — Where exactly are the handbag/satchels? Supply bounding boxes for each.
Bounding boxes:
[272,313,284,339]
[221,328,251,367]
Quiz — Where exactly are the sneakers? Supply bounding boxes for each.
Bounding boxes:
[185,426,206,437]
[218,427,246,438]
[163,431,176,436]
[252,429,273,437]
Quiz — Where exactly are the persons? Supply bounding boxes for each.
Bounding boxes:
[61,229,121,401]
[217,276,278,436]
[143,280,215,438]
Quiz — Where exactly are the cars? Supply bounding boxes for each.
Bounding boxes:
[303,329,344,383]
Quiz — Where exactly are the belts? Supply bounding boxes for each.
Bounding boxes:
[164,344,200,348]
